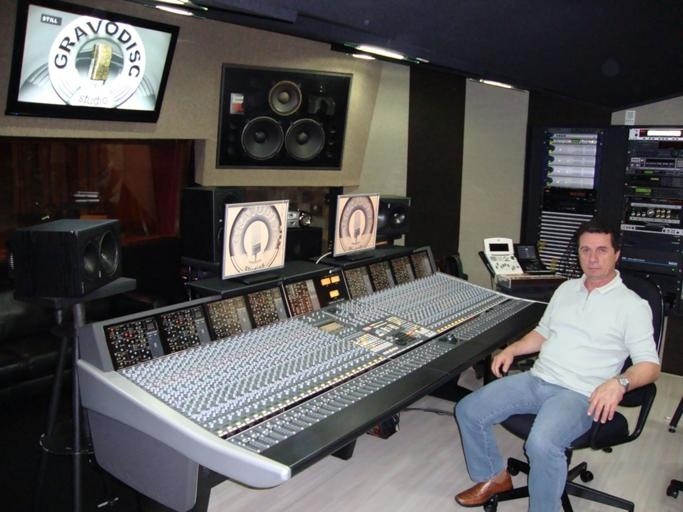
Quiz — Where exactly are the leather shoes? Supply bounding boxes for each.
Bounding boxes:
[455,473,513,508]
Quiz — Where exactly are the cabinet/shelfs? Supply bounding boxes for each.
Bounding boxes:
[12,276,137,512]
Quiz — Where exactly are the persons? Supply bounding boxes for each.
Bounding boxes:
[454,220,661,512]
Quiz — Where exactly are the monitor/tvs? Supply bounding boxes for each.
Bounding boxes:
[5,1,180,124]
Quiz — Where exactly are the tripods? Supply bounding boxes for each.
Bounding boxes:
[35,306,102,512]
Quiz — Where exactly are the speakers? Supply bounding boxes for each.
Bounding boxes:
[13,218,125,299]
[376,193,411,245]
[216,63,354,170]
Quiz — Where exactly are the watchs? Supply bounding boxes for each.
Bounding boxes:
[611,375,630,392]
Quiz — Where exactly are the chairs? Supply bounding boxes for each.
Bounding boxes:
[483,274,669,512]
